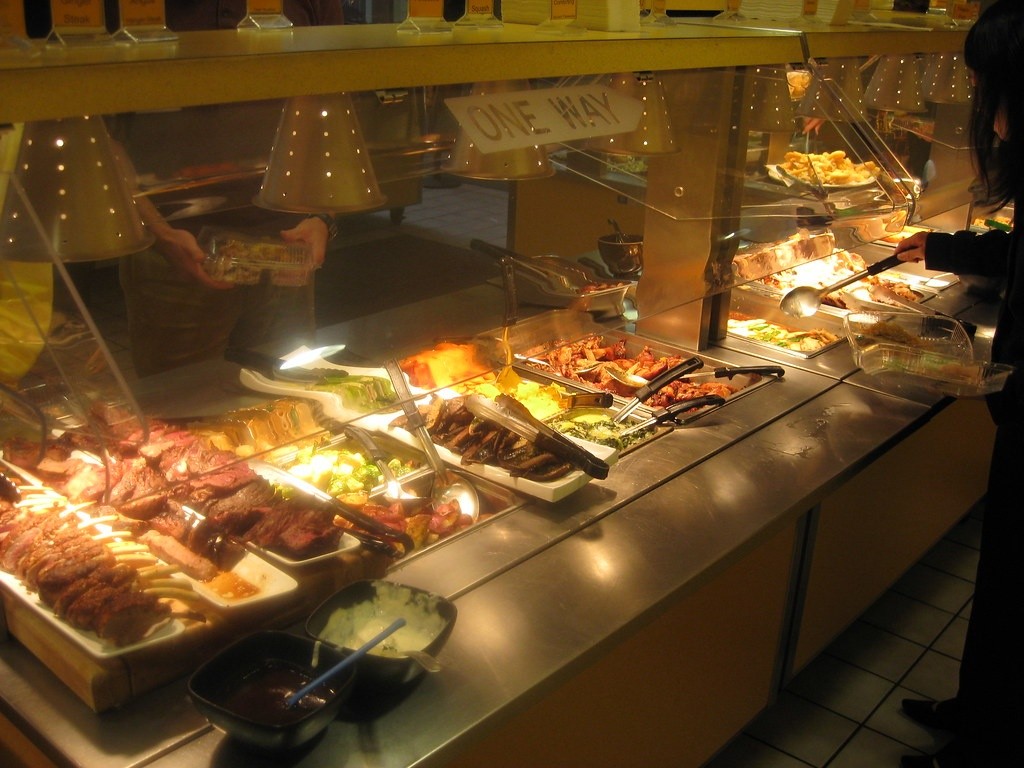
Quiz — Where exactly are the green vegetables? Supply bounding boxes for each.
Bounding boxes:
[727,318,805,348]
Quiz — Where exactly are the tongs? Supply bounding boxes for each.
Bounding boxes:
[840,285,977,339]
[470,239,593,294]
[776,157,829,201]
[247,459,414,559]
[465,393,609,481]
[224,348,348,382]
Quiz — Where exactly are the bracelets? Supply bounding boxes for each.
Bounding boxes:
[308,212,338,242]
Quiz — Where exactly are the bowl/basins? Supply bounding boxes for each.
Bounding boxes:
[843,311,974,370]
[599,234,643,276]
[207,237,310,286]
[155,200,1017,747]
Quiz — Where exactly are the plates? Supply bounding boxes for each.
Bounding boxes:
[240,345,426,425]
[0,566,184,658]
[51,424,361,567]
[381,388,618,502]
[0,451,299,607]
[768,167,876,188]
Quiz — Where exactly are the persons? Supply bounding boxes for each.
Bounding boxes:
[893,0,1024,768]
[111,0,347,374]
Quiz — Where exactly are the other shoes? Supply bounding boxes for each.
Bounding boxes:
[901,698,965,732]
[900,754,935,768]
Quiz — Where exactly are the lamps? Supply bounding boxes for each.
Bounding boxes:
[440,79,556,181]
[585,72,683,157]
[921,53,972,104]
[865,54,929,113]
[796,57,868,122]
[252,92,389,215]
[0,112,156,264]
[748,64,795,133]
